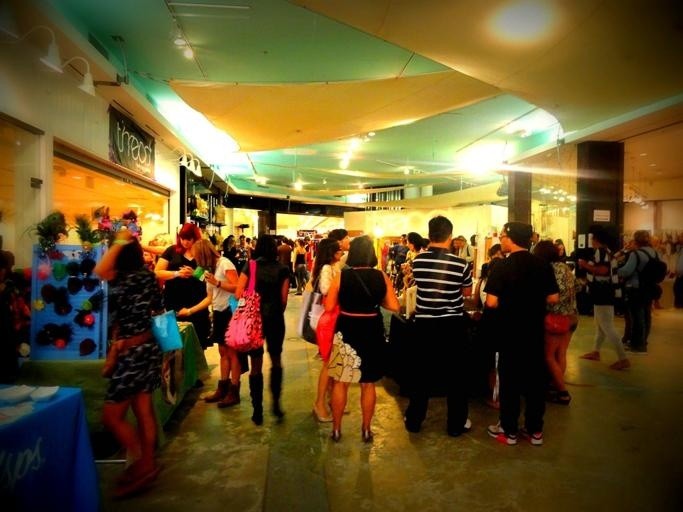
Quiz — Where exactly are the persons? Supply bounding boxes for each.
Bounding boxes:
[575,228,682,370]
[1,215,294,494]
[289,216,576,446]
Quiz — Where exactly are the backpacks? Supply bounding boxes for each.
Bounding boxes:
[638,248,667,285]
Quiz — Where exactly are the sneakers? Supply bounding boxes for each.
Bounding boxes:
[403,415,472,436]
[610,359,631,371]
[621,337,649,357]
[519,426,545,448]
[362,430,374,442]
[487,421,516,446]
[580,350,602,361]
[329,430,342,441]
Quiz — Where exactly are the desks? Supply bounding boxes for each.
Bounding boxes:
[20,321,210,446]
[0,384,101,512]
[380,307,495,400]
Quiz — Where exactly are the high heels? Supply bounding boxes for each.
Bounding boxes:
[328,399,351,416]
[312,406,335,424]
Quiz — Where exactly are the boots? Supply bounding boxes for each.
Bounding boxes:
[268,366,286,420]
[248,373,265,427]
[204,378,232,403]
[217,380,241,409]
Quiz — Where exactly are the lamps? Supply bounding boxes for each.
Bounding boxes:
[0,20,63,76]
[64,57,95,96]
[179,159,202,178]
[174,154,195,173]
[165,148,187,178]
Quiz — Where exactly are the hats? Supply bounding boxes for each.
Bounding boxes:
[504,221,533,251]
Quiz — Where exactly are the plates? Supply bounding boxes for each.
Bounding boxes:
[0,385,60,403]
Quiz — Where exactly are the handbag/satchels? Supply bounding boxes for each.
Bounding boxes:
[297,289,341,365]
[149,308,184,355]
[588,260,617,307]
[224,289,266,352]
